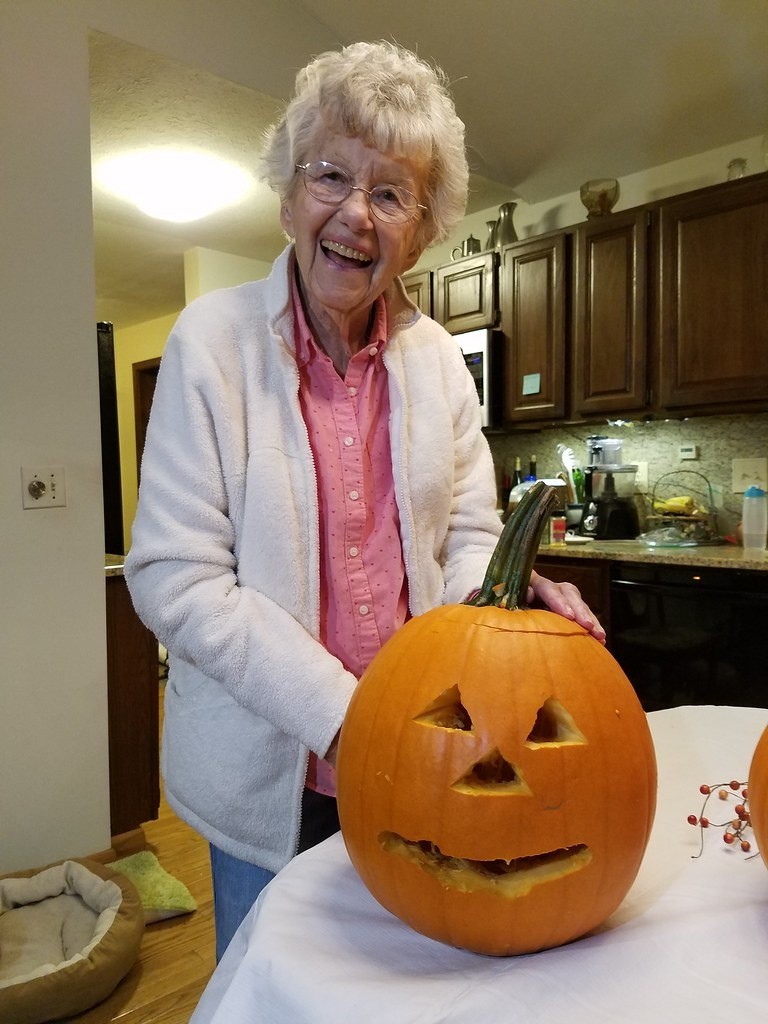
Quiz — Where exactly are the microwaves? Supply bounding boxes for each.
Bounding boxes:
[445,328,495,430]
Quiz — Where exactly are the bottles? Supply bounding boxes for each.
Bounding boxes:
[502,454,538,510]
[742,485,767,552]
[484,202,518,251]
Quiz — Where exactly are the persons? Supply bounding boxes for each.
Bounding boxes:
[122,41,607,966]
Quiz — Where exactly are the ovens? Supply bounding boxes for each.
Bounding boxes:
[607,567,768,712]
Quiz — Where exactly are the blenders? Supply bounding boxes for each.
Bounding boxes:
[578,434,640,541]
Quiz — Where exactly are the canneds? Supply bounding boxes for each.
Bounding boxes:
[550,517,567,546]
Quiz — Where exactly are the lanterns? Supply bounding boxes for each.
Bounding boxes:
[336,604,659,965]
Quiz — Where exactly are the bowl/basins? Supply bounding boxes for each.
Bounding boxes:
[579,178,621,218]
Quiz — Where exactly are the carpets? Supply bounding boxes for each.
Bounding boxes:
[107,850,198,925]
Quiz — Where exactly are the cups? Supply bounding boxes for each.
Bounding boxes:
[726,158,747,181]
[449,233,481,262]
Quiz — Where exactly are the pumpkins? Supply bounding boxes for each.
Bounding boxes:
[748,723,768,873]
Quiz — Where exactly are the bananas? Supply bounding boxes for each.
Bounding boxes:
[644,494,709,517]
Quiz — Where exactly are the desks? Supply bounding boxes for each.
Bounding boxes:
[184,706,768,1022]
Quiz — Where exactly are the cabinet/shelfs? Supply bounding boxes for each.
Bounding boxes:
[500,203,652,436]
[106,563,161,839]
[532,559,615,655]
[655,167,768,417]
[393,244,499,335]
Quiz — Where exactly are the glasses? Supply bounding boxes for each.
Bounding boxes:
[289,153,431,225]
